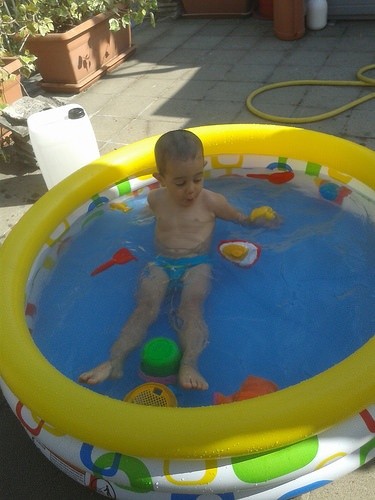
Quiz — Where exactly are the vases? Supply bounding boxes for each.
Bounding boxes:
[181,0,255,16]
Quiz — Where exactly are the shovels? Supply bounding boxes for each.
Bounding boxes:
[90,248,136,275]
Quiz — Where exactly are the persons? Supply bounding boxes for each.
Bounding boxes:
[78,129,284,392]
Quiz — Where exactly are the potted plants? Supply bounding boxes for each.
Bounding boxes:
[0,1,158,93]
[0,46,38,138]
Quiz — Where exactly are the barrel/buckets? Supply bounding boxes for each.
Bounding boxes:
[306,0,328,31]
[26,103,101,191]
